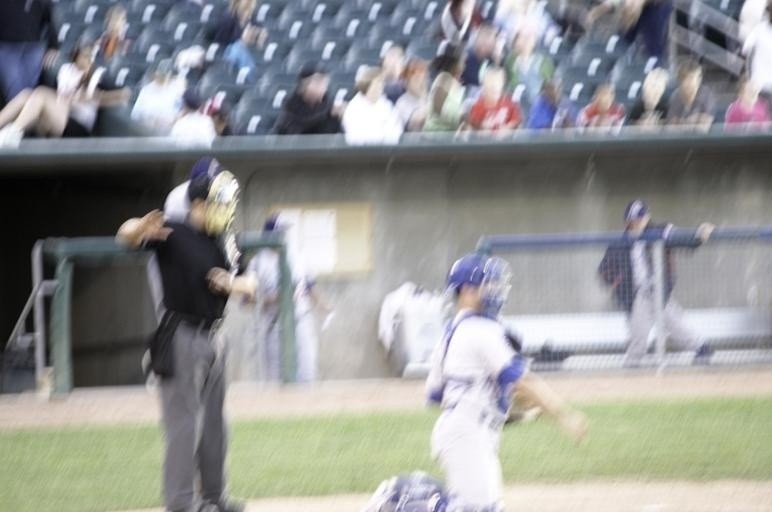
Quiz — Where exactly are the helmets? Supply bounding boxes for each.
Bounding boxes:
[446,251,500,284]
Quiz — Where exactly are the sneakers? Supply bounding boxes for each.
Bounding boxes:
[178,489,247,512]
[376,470,438,512]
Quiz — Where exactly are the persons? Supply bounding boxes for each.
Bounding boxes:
[360,247,599,512]
[144,158,228,328]
[1,0,772,150]
[597,198,719,369]
[114,163,266,511]
[242,210,336,384]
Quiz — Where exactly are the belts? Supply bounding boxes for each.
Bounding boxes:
[176,310,225,332]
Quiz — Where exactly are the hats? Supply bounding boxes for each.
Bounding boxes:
[623,200,650,218]
